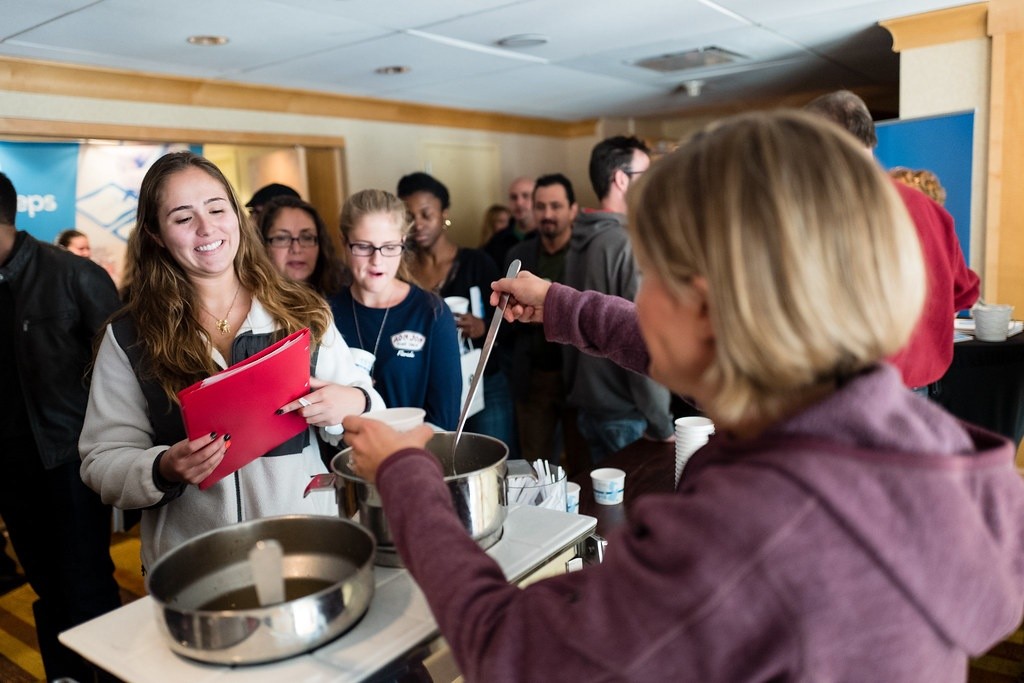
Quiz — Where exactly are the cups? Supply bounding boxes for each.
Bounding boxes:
[591,468,627,505]
[445,295,470,320]
[359,408,427,508]
[971,304,1015,342]
[350,347,376,373]
[566,480,581,513]
[673,417,716,493]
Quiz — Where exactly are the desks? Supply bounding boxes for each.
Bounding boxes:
[926,316,1024,460]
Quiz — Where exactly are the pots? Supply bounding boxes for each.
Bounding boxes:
[304,431,537,569]
[144,515,376,666]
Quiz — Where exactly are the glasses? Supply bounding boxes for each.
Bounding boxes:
[345,232,407,257]
[264,235,322,248]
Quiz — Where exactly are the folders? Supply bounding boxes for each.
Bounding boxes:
[177,328,311,494]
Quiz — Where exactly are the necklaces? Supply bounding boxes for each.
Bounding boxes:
[352,293,394,379]
[198,282,241,336]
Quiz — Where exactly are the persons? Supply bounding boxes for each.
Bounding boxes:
[343,110,1024,683]
[562,134,676,454]
[804,90,982,401]
[58,229,91,259]
[481,173,578,461]
[396,171,514,447]
[77,152,386,575]
[0,173,123,683]
[324,190,463,431]
[245,184,341,299]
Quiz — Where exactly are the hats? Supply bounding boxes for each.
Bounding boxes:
[246,185,298,208]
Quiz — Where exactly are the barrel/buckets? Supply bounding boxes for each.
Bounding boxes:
[508,461,567,514]
